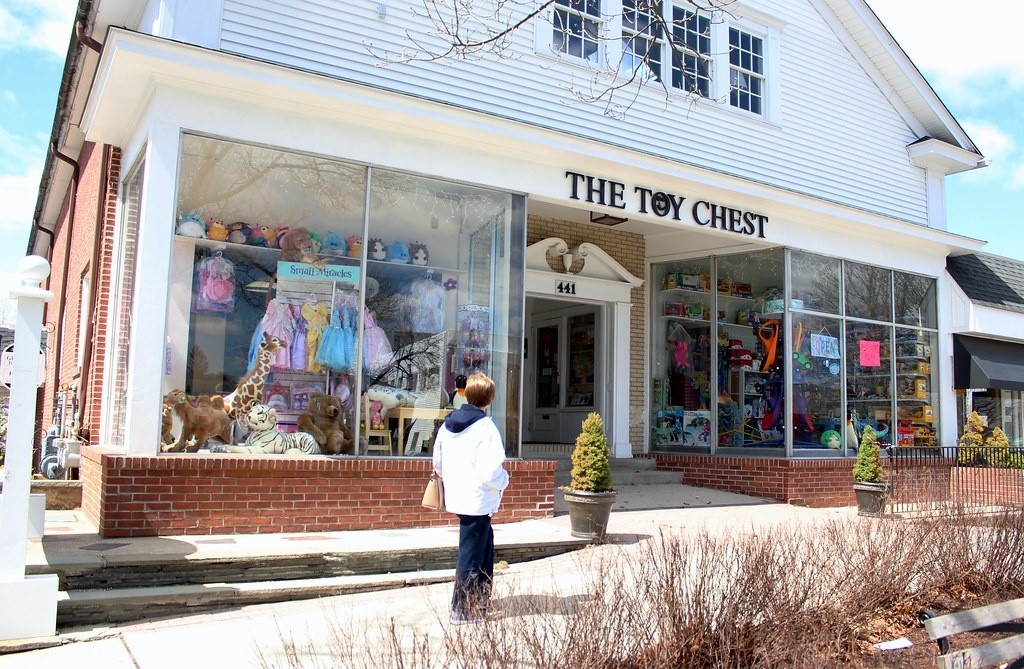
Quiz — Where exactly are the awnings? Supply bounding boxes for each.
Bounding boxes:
[953,332,1024,392]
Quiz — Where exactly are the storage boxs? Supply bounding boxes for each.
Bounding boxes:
[683,411,710,446]
[883,343,934,446]
[728,339,752,370]
[662,271,762,327]
[651,411,684,445]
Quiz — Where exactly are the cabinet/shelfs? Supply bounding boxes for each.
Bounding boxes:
[727,370,782,446]
[824,317,934,446]
[659,288,824,333]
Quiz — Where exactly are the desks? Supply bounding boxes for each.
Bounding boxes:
[384,406,453,456]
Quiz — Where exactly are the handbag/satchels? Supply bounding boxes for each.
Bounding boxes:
[421,468,443,512]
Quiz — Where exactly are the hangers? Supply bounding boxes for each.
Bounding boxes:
[400,274,442,293]
[195,250,235,270]
[275,293,367,311]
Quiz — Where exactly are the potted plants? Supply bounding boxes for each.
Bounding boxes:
[558,412,619,540]
[852,424,890,517]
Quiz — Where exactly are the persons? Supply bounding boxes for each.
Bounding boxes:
[670,417,682,442]
[433,372,509,627]
[698,420,710,443]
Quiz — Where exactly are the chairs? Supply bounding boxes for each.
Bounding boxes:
[360,396,393,456]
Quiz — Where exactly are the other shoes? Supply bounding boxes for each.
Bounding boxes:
[450,609,487,624]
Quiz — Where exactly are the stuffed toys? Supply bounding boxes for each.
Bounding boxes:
[253,223,278,249]
[325,232,348,256]
[856,418,889,441]
[225,221,251,244]
[847,412,859,453]
[264,226,335,311]
[450,374,471,410]
[175,210,210,240]
[206,217,231,242]
[223,331,288,420]
[210,404,321,455]
[160,389,238,453]
[343,235,363,258]
[409,241,429,266]
[367,238,388,260]
[821,430,841,449]
[297,392,369,456]
[386,239,411,263]
